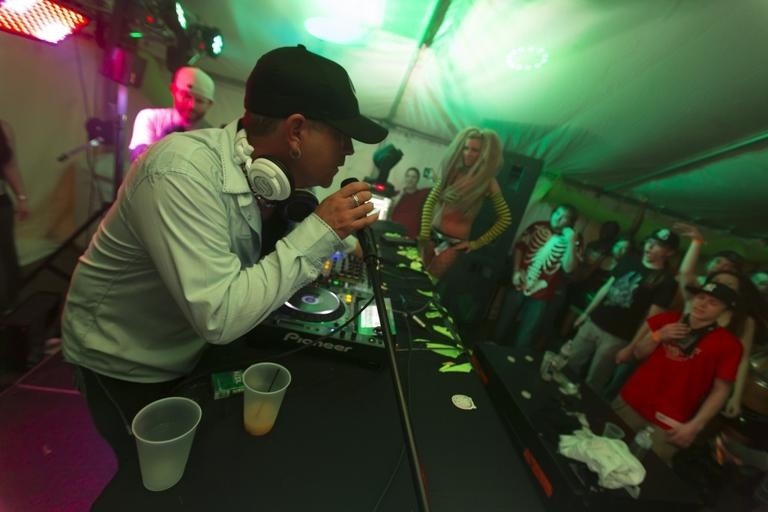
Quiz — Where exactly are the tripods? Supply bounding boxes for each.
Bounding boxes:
[12,89,138,300]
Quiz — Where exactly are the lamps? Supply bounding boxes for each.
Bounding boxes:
[185,23,223,58]
[127,0,188,41]
[0,1,92,47]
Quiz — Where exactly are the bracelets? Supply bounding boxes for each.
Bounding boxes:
[16,193,28,201]
[652,331,662,343]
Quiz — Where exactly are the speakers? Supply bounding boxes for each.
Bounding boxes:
[441,151,545,332]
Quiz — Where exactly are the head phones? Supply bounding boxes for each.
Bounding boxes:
[233,117,321,223]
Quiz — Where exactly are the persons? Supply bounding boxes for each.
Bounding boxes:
[499,203,767,464]
[61,44,389,459]
[389,167,438,240]
[127,64,215,164]
[415,125,514,284]
[415,127,513,284]
[0,120,32,294]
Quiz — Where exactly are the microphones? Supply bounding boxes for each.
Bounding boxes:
[339,176,384,271]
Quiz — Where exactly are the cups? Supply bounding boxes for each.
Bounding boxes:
[242,362,291,436]
[603,422,625,440]
[540,350,556,372]
[131,397,202,492]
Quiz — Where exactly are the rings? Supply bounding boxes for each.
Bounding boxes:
[350,192,361,208]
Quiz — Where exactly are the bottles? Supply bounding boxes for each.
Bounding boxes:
[629,426,655,461]
[542,360,556,382]
[559,340,572,370]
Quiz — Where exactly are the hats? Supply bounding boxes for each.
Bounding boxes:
[244,45,388,143]
[176,67,215,103]
[645,229,678,249]
[686,282,734,307]
